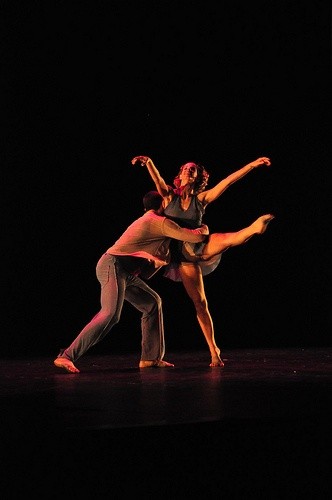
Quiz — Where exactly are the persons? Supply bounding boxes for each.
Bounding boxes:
[51,192,210,374]
[130,152,273,366]
